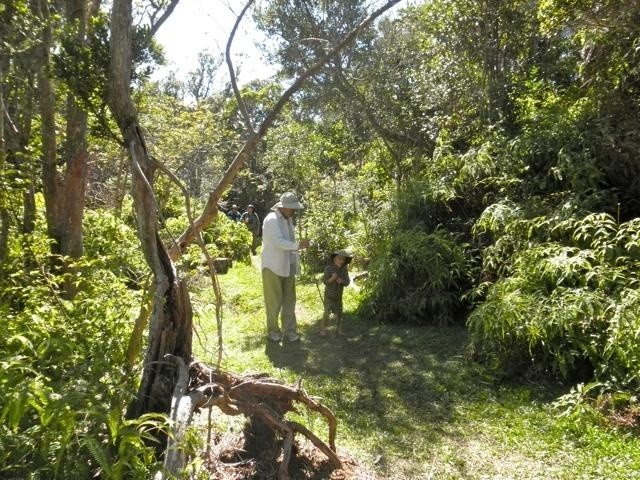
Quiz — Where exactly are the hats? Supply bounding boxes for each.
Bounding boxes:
[271,191,304,211]
[331,250,352,264]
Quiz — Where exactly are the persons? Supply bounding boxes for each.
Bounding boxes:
[260,192,309,344]
[318,250,353,337]
[240,204,259,257]
[224,200,241,222]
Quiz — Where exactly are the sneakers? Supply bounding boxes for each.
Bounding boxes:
[269,331,300,342]
